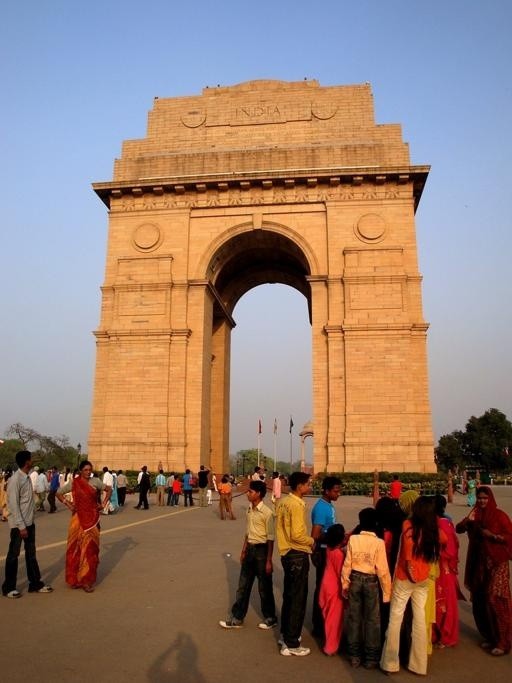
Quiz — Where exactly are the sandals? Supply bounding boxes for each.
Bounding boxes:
[70,584,82,589]
[82,584,95,593]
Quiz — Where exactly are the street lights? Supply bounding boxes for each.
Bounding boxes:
[76,441,84,469]
[242,453,245,477]
[236,460,240,481]
[262,455,267,470]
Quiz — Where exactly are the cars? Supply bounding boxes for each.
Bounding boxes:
[507,474,511,480]
[488,473,496,479]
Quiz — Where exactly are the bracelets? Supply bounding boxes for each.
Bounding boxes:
[63,499,67,504]
[101,503,105,507]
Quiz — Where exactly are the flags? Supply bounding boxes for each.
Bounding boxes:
[289,416,294,433]
[259,417,262,433]
[273,417,278,434]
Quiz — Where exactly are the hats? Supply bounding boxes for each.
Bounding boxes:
[34,466,39,471]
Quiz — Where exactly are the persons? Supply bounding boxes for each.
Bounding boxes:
[1,450,53,599]
[455,486,510,657]
[341,490,453,667]
[310,476,350,647]
[272,472,281,507]
[219,477,237,520]
[467,474,476,507]
[276,472,317,657]
[380,496,448,675]
[390,474,403,500]
[340,510,392,670]
[223,474,232,516]
[219,480,278,630]
[55,460,113,594]
[431,494,460,649]
[1,460,266,524]
[318,523,351,657]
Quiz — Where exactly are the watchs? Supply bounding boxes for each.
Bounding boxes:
[491,535,495,542]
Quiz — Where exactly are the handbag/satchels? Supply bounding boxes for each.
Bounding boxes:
[189,478,198,488]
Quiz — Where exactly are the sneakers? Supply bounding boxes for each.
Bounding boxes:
[36,586,54,593]
[434,640,450,649]
[365,661,378,671]
[280,642,312,657]
[256,615,279,630]
[407,669,422,676]
[379,667,393,677]
[480,641,491,649]
[351,657,361,668]
[6,589,22,599]
[218,615,245,629]
[277,633,302,646]
[491,647,507,656]
